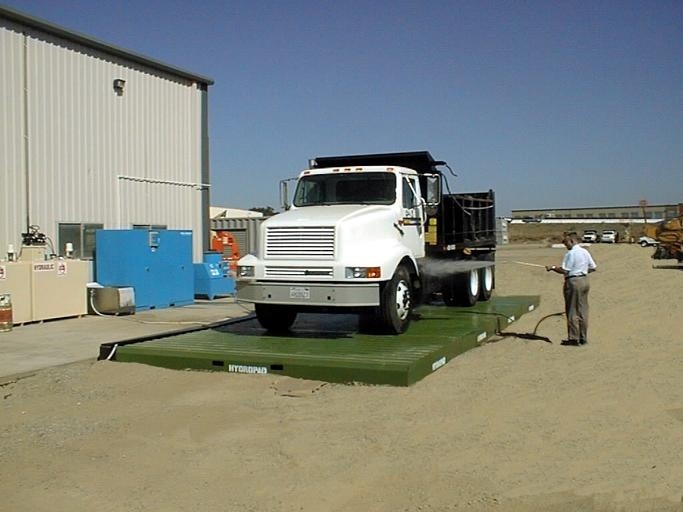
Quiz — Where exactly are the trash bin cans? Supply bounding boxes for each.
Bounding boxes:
[0,294,13,333]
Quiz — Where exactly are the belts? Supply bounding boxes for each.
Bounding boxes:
[565,275,586,281]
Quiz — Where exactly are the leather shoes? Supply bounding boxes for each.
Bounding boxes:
[561,339,585,346]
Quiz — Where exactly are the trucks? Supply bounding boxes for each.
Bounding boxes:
[581,229,619,243]
[235,151,496,335]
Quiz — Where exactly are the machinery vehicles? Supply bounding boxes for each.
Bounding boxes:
[621,200,683,269]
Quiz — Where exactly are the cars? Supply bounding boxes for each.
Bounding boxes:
[523,216,541,223]
[501,217,512,221]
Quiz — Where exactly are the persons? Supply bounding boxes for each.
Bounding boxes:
[544,232,596,346]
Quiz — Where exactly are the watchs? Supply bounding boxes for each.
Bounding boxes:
[551,264,556,270]
